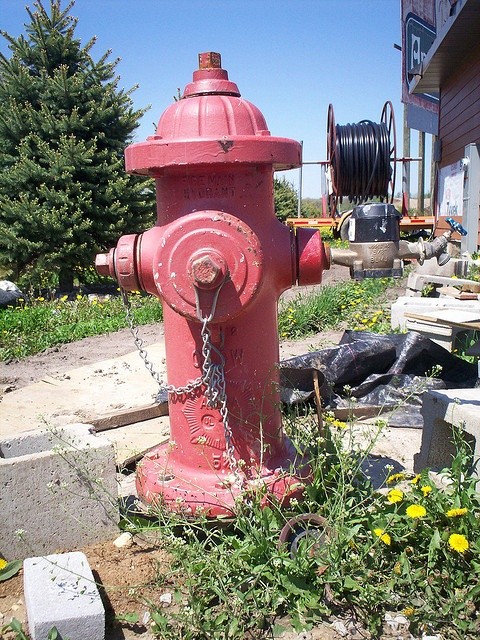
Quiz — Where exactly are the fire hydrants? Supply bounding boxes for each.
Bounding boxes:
[96,52,331,525]
